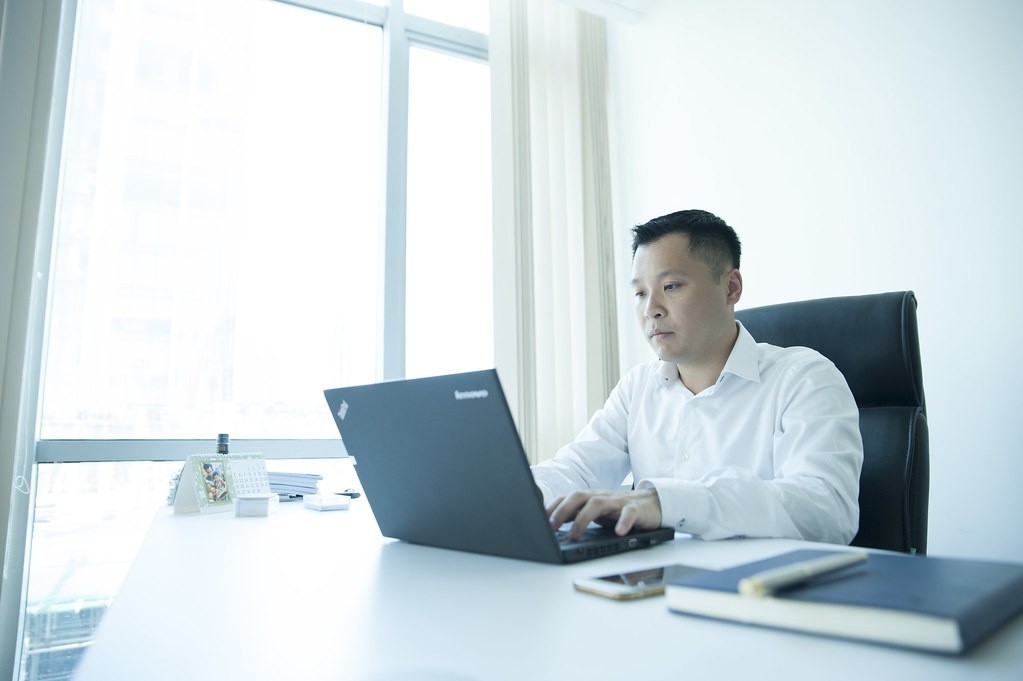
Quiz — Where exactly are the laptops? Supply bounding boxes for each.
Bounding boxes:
[323,369,675,565]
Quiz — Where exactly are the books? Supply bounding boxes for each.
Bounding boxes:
[663,550,1023,657]
[266,470,324,496]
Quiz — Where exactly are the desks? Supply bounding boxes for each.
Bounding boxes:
[64,498,1022,679]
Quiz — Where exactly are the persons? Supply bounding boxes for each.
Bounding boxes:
[210,471,229,502]
[523,208,863,548]
[202,463,227,499]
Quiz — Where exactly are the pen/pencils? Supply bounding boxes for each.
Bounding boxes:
[741,554,868,596]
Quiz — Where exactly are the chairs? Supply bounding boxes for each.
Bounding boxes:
[733,289,931,558]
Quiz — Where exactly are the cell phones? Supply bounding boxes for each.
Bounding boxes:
[573,565,666,601]
